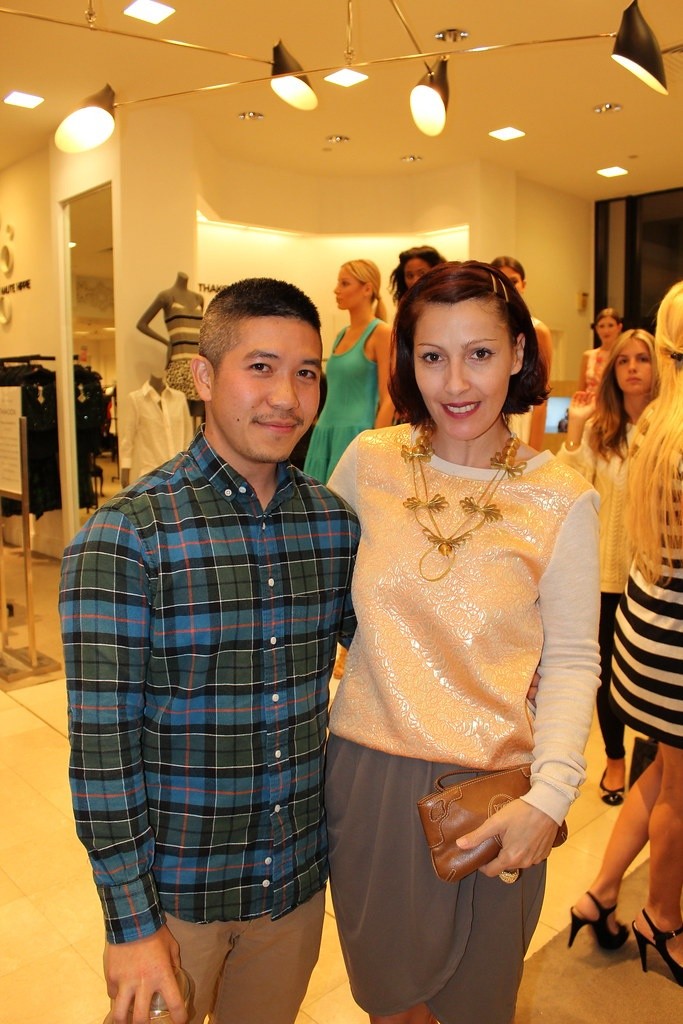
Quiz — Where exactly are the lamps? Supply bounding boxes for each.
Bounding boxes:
[55,83,117,152]
[408,54,450,138]
[270,40,318,111]
[611,1,668,97]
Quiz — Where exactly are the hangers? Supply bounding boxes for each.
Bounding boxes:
[0,357,53,385]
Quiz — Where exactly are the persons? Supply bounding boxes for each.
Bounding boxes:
[56,272,540,1024]
[115,372,194,486]
[316,257,603,1024]
[301,244,552,679]
[136,269,208,437]
[135,268,206,434]
[558,280,683,987]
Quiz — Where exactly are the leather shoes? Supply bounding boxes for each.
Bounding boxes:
[599,768,625,804]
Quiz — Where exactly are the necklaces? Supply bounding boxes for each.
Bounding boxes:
[402,414,527,582]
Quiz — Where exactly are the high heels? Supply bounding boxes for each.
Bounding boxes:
[631,908,683,983]
[566,890,628,952]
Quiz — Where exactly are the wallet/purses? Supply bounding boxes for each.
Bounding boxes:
[416,763,567,883]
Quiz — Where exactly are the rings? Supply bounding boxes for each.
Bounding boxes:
[498,869,519,883]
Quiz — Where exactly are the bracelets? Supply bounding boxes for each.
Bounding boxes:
[565,438,581,449]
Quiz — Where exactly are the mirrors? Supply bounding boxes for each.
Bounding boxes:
[58,183,119,530]
